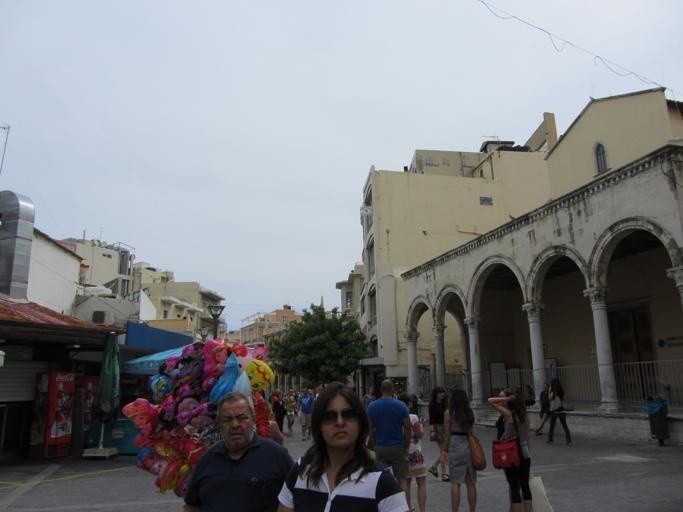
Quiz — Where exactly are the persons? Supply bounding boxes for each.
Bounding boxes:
[265,401,285,445]
[546,378,572,445]
[534,381,550,436]
[441,387,477,512]
[367,380,411,494]
[361,383,377,411]
[272,382,409,512]
[427,387,451,483]
[411,393,420,414]
[299,387,315,441]
[261,389,267,401]
[284,389,299,432]
[522,383,536,407]
[487,389,536,512]
[400,393,428,512]
[182,392,296,512]
[271,393,286,433]
[315,385,324,397]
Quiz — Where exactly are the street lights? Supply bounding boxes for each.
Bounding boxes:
[207,305,225,339]
[196,326,212,341]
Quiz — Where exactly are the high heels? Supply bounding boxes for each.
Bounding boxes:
[566,442,573,447]
[545,439,553,443]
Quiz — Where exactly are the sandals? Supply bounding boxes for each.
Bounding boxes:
[427,465,438,477]
[442,473,451,482]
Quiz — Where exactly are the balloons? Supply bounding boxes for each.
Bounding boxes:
[120,337,273,499]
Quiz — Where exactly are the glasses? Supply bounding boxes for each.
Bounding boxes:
[321,409,358,424]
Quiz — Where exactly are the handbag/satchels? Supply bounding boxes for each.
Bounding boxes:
[411,420,423,441]
[493,410,521,467]
[469,431,487,469]
